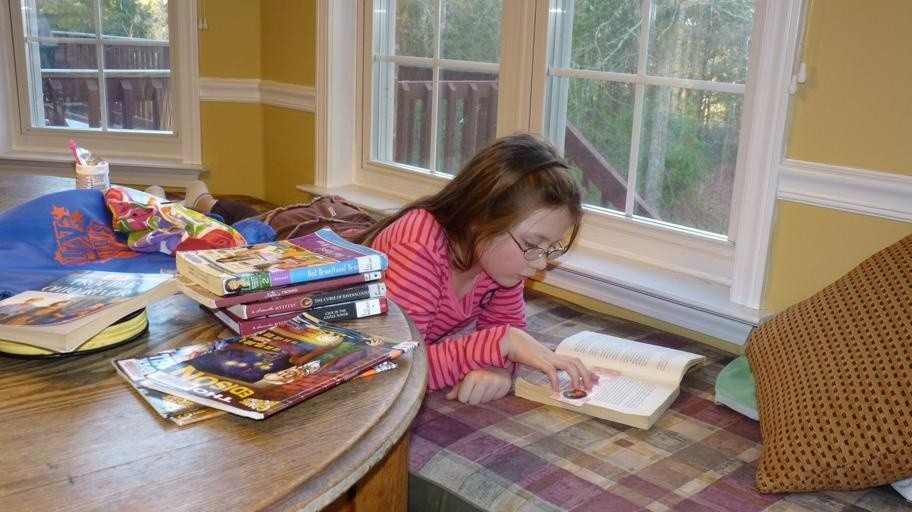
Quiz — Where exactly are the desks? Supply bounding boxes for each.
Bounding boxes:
[4,171,428,511]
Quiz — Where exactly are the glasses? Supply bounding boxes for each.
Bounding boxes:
[505,226,565,261]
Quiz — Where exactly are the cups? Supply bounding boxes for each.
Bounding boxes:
[76,162,109,195]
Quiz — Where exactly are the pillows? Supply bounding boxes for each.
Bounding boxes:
[747,236,911,495]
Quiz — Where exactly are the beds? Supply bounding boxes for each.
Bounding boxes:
[403,282,909,512]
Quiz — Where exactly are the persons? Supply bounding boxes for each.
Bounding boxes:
[144,134,600,405]
[215,248,275,263]
[225,277,249,292]
[1,297,102,325]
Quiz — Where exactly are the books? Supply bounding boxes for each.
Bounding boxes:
[142,312,417,420]
[515,330,707,431]
[111,338,399,428]
[1,269,179,354]
[176,226,388,334]
